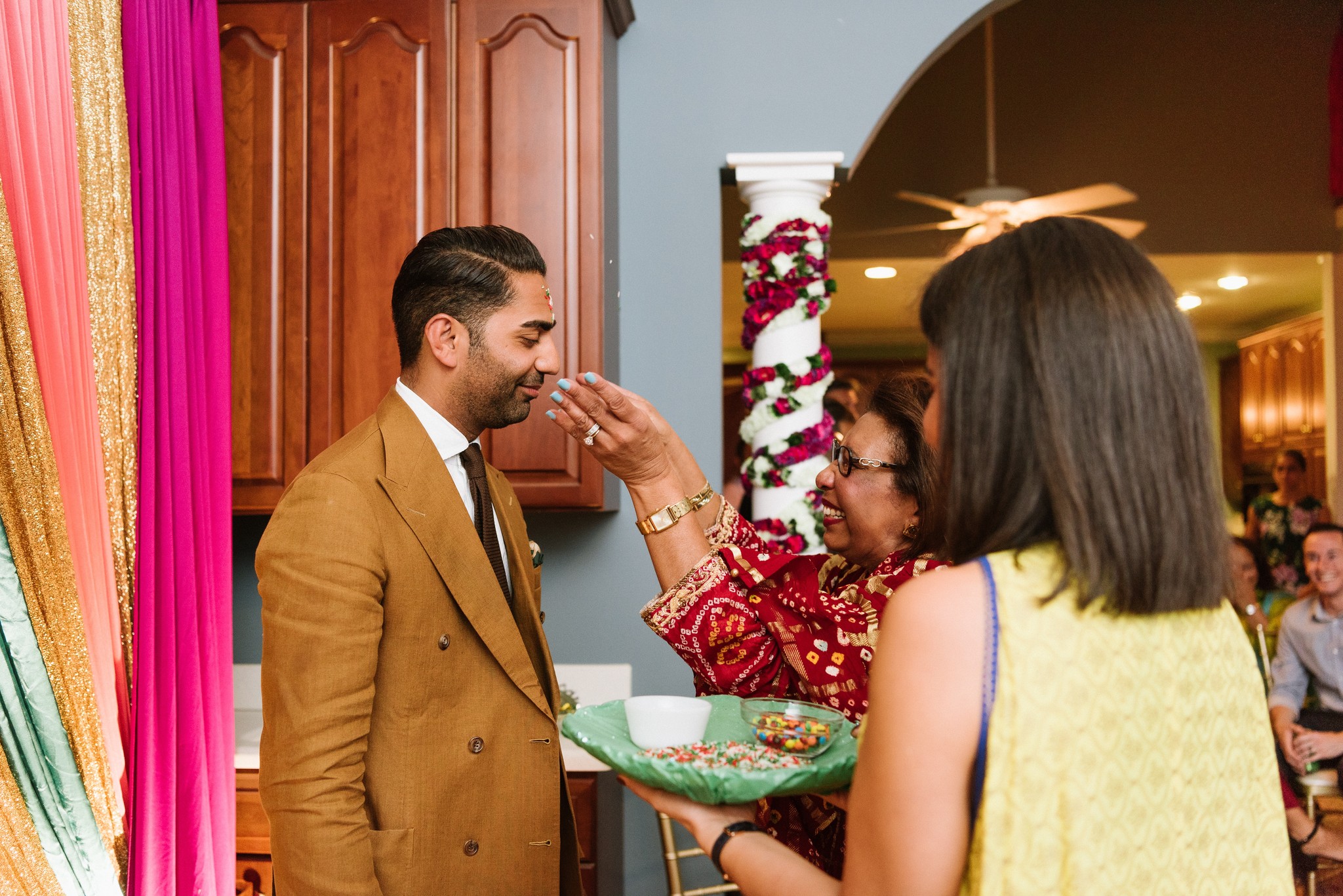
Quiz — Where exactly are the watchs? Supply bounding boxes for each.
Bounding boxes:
[1238,601,1261,616]
[712,820,770,882]
[635,495,694,535]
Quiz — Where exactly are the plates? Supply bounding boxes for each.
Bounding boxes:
[560,688,861,807]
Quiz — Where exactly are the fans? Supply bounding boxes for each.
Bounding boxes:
[846,12,1149,261]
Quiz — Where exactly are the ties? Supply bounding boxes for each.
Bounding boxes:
[459,442,513,615]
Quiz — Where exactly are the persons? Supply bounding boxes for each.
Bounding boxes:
[610,216,1301,896]
[1267,525,1343,876]
[1246,448,1332,600]
[826,379,864,423]
[1223,539,1295,677]
[543,370,941,896]
[820,399,852,441]
[249,226,595,895]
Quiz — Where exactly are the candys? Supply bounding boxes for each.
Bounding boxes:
[636,712,830,771]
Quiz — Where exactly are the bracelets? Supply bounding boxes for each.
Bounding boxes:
[688,479,714,513]
[1299,822,1320,846]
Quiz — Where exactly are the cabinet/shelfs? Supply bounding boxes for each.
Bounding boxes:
[205,0,642,521]
[215,659,635,896]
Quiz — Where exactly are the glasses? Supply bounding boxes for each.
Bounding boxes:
[1277,464,1300,473]
[829,437,912,477]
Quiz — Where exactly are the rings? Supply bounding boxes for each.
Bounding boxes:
[585,423,600,437]
[583,428,602,446]
[1309,747,1314,755]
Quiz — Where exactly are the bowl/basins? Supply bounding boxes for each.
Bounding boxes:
[624,691,712,756]
[739,697,846,758]
